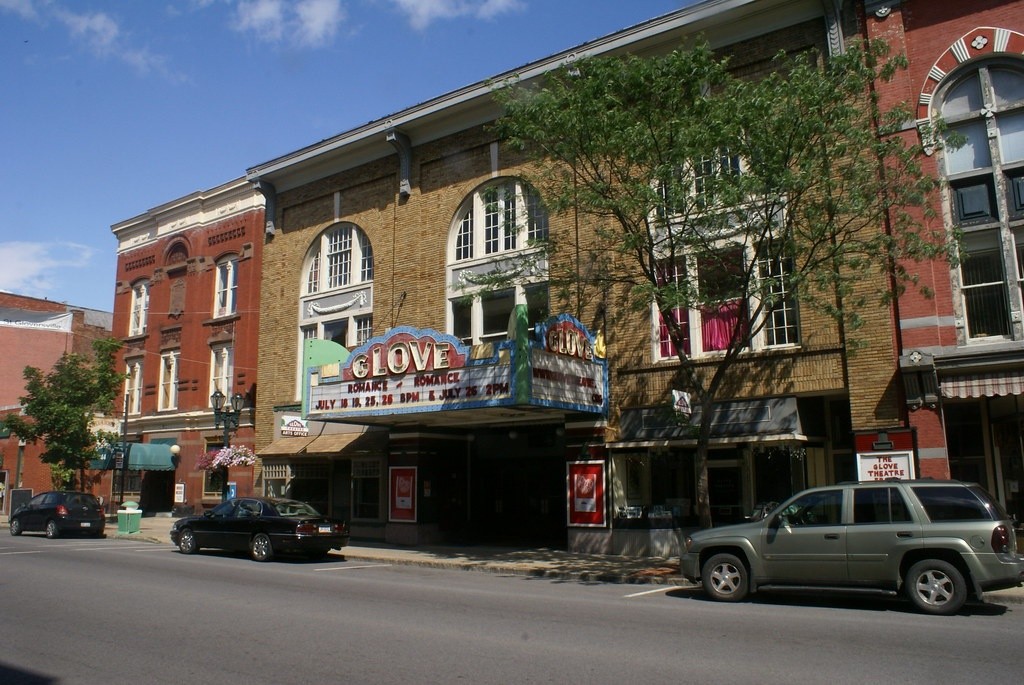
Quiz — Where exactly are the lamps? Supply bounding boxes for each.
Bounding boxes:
[171,444,182,459]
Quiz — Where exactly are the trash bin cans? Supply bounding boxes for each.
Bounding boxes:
[116,501,143,535]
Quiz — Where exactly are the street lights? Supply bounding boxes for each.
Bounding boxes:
[208,388,245,503]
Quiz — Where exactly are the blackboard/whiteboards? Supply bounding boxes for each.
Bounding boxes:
[9,488,34,523]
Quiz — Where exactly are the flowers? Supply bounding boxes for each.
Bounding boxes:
[194,444,261,472]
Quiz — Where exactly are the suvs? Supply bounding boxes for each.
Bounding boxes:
[678,474,1023,614]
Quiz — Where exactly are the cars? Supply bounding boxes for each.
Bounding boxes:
[169,498,351,565]
[7,491,108,539]
